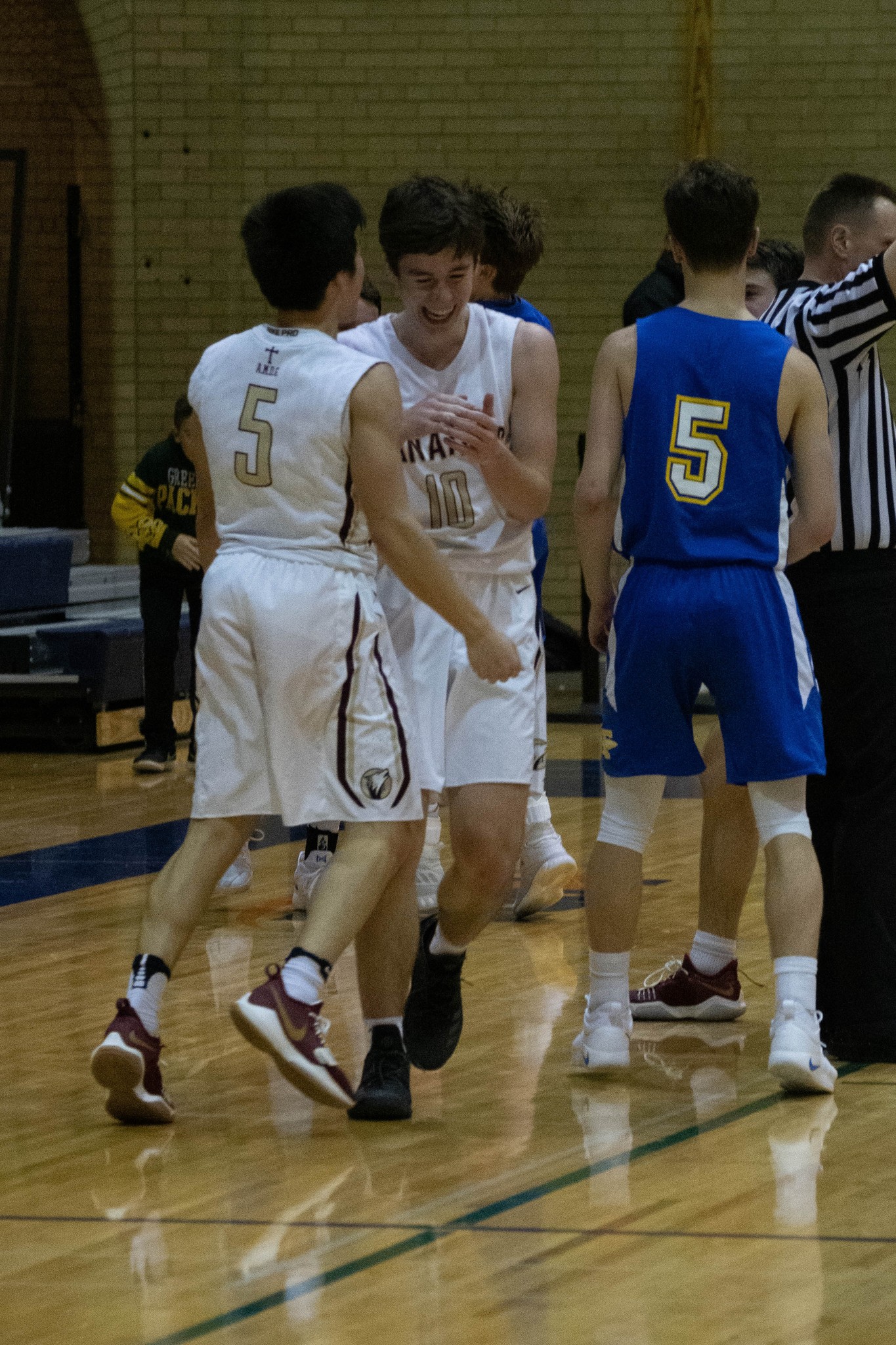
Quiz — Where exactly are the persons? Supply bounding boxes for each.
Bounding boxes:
[89,160,896,1128]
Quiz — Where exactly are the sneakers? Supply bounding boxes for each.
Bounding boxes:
[628,1023,745,1079]
[219,1132,358,1275]
[414,840,446,909]
[571,995,631,1068]
[345,1024,412,1121]
[89,996,174,1121]
[230,962,357,1108]
[205,929,254,993]
[292,849,333,909]
[186,745,197,768]
[509,920,576,1022]
[761,1093,838,1177]
[133,749,176,770]
[403,913,467,1070]
[572,1079,634,1159]
[767,1000,838,1091]
[510,835,576,919]
[629,951,749,1020]
[131,773,168,789]
[217,828,265,891]
[90,1123,175,1220]
